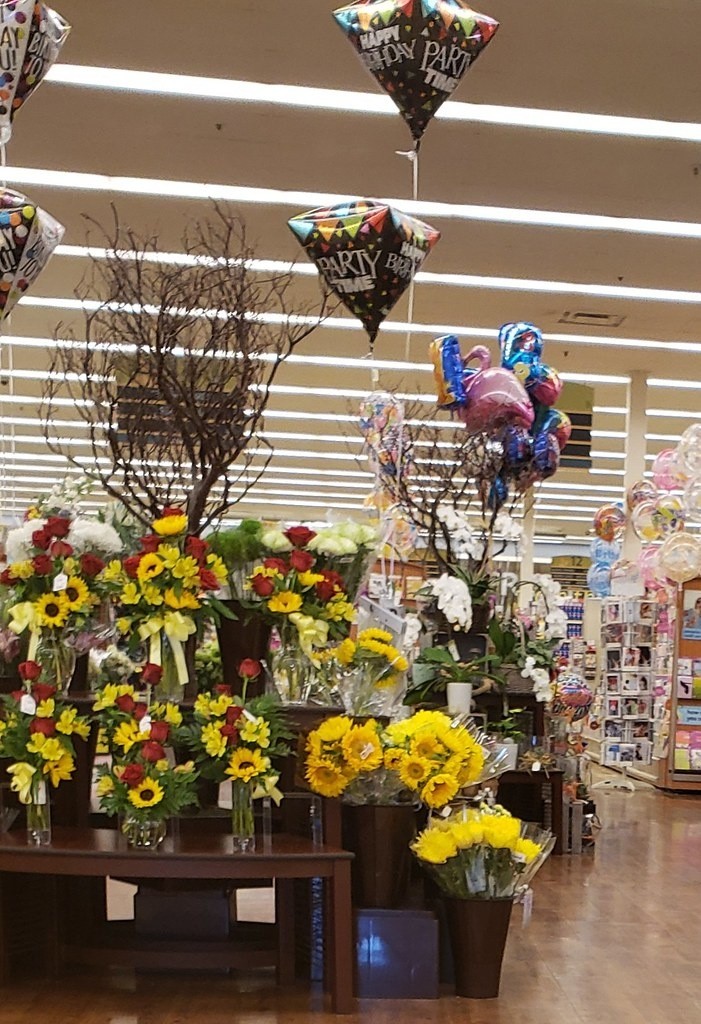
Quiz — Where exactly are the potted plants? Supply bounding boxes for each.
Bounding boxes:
[406,644,505,714]
[486,707,526,771]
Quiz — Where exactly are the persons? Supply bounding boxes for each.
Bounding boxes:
[685,598,701,628]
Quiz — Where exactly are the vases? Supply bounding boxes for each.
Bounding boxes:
[230,779,257,850]
[343,801,418,909]
[146,632,187,703]
[121,811,167,851]
[26,783,51,846]
[271,625,316,705]
[442,894,514,1000]
[34,636,76,696]
[213,599,283,698]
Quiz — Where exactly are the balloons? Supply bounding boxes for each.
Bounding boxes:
[429,323,570,510]
[360,390,420,558]
[332,0,500,144]
[0,0,72,124]
[0,186,66,322]
[587,423,701,595]
[287,201,440,343]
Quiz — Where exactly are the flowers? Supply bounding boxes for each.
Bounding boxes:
[407,803,545,896]
[0,473,569,833]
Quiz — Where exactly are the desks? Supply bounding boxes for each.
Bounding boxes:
[498,767,567,856]
[0,826,356,1014]
[0,691,346,987]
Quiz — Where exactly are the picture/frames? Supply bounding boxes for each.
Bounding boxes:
[591,598,661,791]
